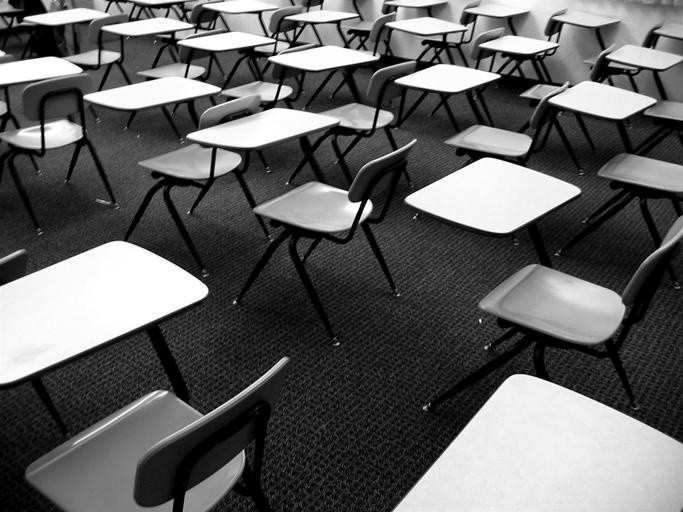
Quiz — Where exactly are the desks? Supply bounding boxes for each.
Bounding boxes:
[186,107,341,187]
[548,80,658,154]
[391,374,681,510]
[405,156,582,270]
[606,43,683,100]
[0,239,210,399]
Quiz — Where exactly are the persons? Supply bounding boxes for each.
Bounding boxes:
[7,0,53,58]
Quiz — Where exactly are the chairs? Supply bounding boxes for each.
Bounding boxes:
[233,138,417,347]
[0,248,67,432]
[0,1,620,133]
[584,21,667,100]
[555,151,683,291]
[518,43,617,176]
[0,53,42,177]
[635,100,682,156]
[0,72,120,237]
[123,93,274,278]
[24,356,289,512]
[422,214,683,413]
[443,81,571,167]
